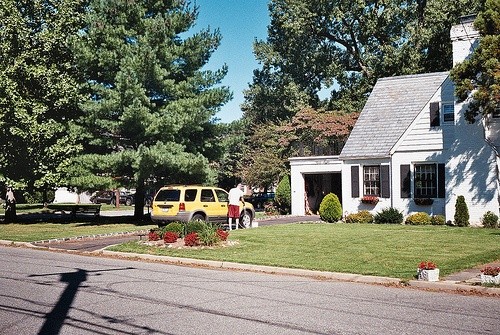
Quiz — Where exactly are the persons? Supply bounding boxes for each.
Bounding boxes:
[228,183,245,231]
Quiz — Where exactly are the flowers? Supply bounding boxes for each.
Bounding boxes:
[480,265,500,276]
[361,196,378,200]
[419,262,436,270]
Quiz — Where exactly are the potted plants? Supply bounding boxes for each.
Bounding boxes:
[480,273,500,286]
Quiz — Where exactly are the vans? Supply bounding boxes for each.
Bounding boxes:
[151,185,255,229]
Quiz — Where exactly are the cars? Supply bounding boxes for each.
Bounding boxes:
[90,191,153,207]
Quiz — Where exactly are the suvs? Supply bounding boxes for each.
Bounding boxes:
[243,192,275,212]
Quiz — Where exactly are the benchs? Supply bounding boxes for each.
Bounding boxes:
[41,205,102,225]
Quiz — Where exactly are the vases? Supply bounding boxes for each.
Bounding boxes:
[416,266,439,283]
[413,197,434,205]
[360,199,379,205]
